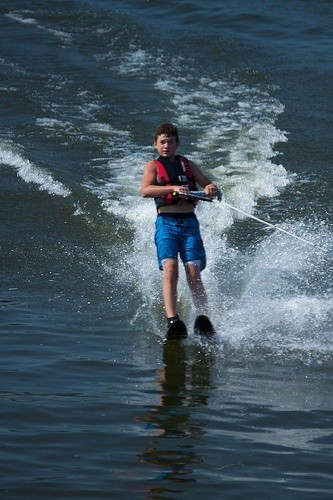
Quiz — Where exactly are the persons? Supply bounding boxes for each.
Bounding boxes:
[140,122,221,338]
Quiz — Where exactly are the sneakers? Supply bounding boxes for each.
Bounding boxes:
[193,315,215,338]
[164,314,188,341]
